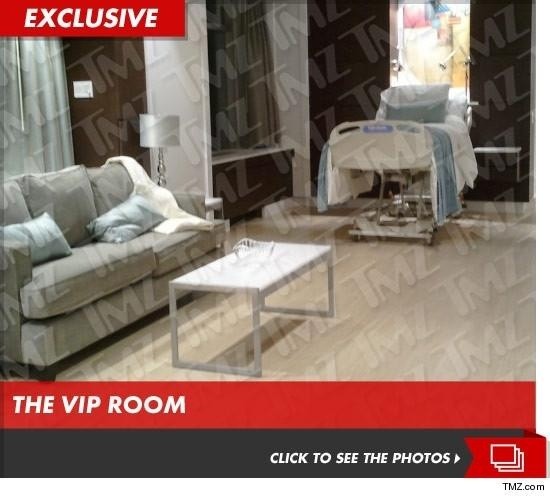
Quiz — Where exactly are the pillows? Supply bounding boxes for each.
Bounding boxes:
[0,199,166,266]
[375,85,469,127]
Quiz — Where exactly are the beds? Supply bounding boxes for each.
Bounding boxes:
[327,83,473,244]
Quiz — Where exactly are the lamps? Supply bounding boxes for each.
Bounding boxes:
[139,114,180,186]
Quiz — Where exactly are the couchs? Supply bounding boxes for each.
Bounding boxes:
[0,161,232,383]
